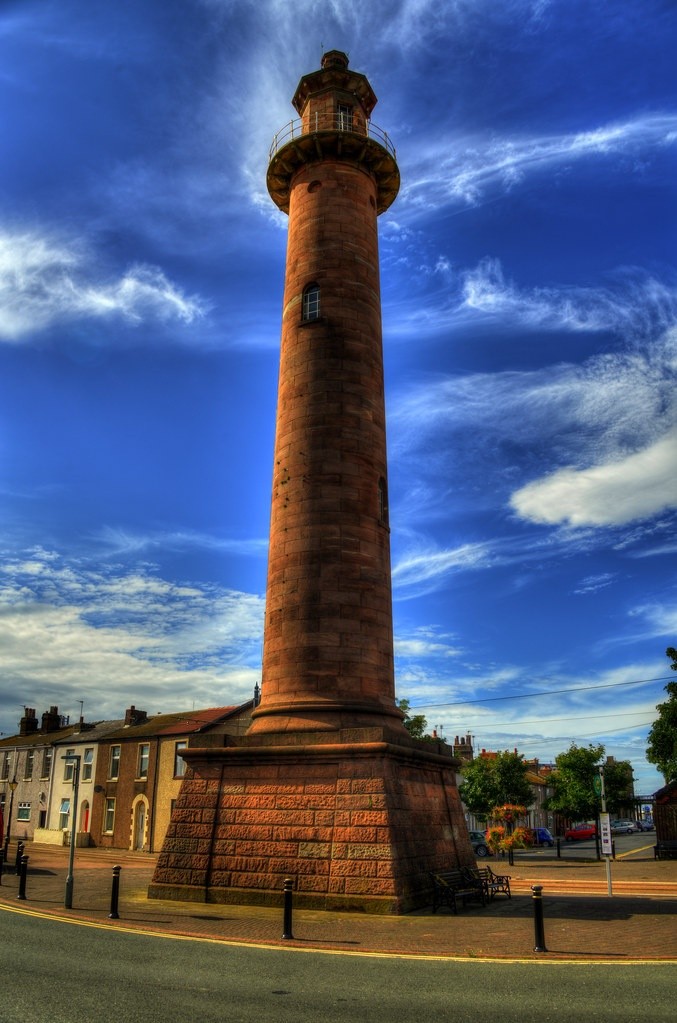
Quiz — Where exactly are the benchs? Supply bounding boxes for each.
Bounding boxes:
[430,868,483,915]
[470,865,512,903]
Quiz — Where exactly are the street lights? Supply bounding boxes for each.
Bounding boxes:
[5,775,20,863]
[60,755,82,914]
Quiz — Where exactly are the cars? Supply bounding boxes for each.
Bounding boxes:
[606,816,655,834]
[565,823,597,842]
[528,828,554,848]
[465,830,491,857]
